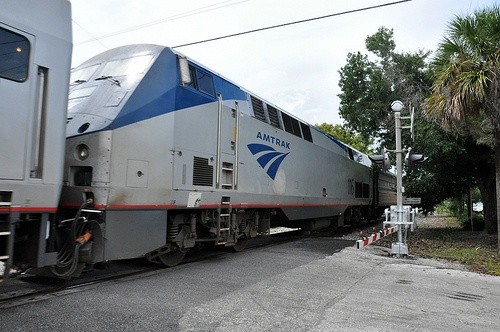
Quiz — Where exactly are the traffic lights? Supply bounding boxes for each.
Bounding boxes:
[404,146,430,170]
[368,153,390,170]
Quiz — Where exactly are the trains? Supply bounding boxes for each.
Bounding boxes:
[0,1,398,283]
